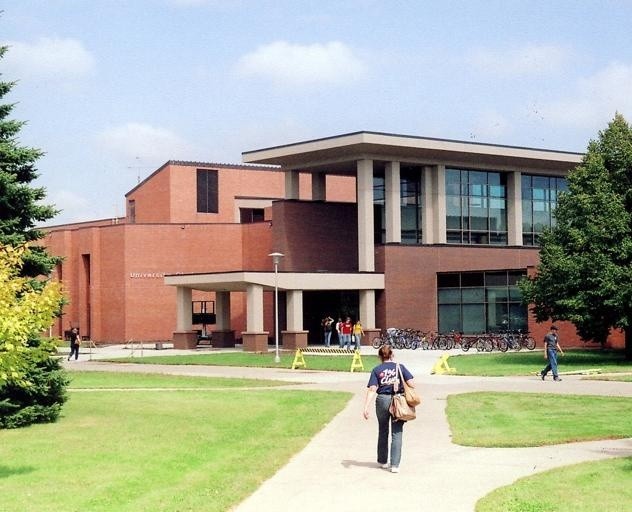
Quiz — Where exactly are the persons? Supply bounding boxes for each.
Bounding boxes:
[364,343,414,472]
[540,325,565,381]
[321,315,366,352]
[68,327,81,362]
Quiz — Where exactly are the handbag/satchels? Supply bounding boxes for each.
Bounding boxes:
[387,392,418,423]
[397,361,421,408]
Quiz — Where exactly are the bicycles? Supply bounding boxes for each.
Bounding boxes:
[370,326,538,353]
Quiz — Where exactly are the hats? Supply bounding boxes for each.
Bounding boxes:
[550,325,560,332]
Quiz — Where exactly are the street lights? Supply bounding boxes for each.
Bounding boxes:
[267,250,286,362]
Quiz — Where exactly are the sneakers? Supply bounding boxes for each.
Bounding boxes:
[390,465,399,473]
[325,345,361,350]
[67,357,78,361]
[540,372,544,380]
[553,378,562,381]
[377,462,389,468]
